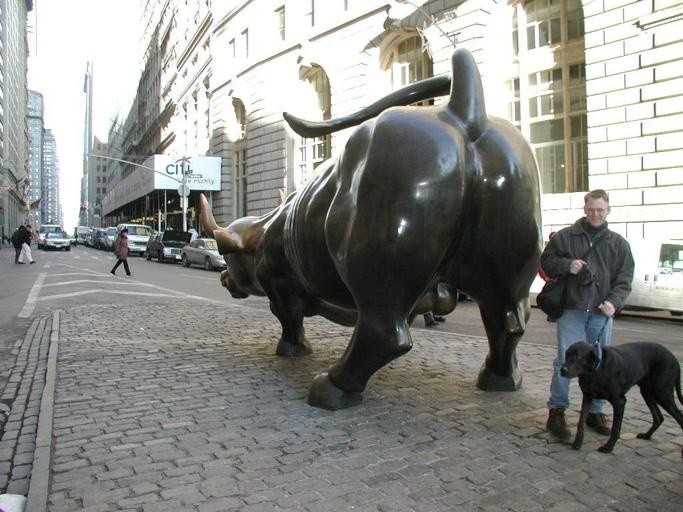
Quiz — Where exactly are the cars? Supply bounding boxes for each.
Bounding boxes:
[35,222,154,256]
[145,230,191,263]
[179,238,227,271]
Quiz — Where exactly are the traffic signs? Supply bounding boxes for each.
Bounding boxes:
[184,170,193,174]
[184,174,202,178]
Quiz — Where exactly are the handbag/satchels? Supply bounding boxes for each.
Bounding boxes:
[537,273,570,321]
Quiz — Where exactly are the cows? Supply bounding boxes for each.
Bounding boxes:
[199,47,544,411]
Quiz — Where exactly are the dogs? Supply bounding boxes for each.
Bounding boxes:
[559,341,683,458]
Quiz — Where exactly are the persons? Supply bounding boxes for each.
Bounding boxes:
[18,225,35,264]
[11,226,25,263]
[111,229,131,277]
[540,189,635,438]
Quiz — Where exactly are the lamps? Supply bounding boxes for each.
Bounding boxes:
[395,0,456,50]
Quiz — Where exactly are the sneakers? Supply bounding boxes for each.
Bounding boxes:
[30,260,38,265]
[125,274,133,278]
[424,318,440,327]
[109,272,116,278]
[547,407,572,441]
[15,261,26,265]
[583,412,612,435]
